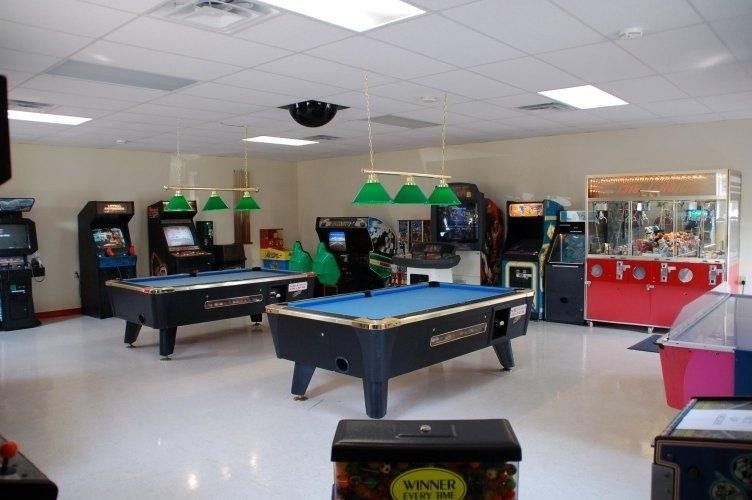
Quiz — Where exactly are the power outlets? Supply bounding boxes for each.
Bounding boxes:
[739,275,747,286]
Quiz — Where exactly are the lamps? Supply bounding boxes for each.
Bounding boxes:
[350,74,463,207]
[163,119,262,213]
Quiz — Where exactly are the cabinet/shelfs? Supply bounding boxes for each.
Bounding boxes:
[584,168,742,335]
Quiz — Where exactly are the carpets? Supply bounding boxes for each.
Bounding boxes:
[626,334,663,354]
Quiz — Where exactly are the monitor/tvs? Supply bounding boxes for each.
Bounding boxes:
[162,225,196,247]
[549,232,586,265]
[328,231,347,252]
[506,213,545,250]
[91,228,127,250]
[0,223,32,252]
[431,192,486,251]
[687,210,710,222]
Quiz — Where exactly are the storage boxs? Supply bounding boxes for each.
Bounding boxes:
[329,416,522,500]
